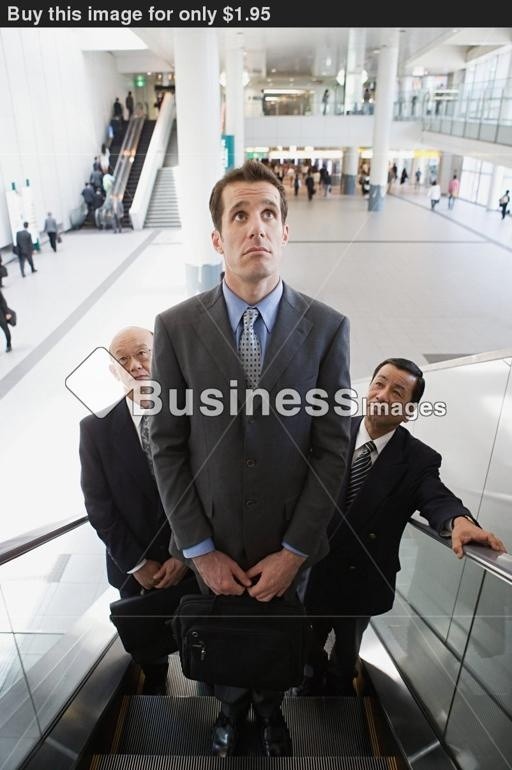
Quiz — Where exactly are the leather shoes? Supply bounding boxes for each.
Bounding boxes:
[143,669,166,696]
[193,677,213,695]
[332,660,358,695]
[294,671,317,696]
[259,713,293,757]
[212,712,239,756]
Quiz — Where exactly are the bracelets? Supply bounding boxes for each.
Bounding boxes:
[451,515,476,530]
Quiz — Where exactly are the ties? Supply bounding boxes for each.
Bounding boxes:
[244,309,262,389]
[141,414,154,482]
[342,442,376,506]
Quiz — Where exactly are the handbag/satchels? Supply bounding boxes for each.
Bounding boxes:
[109,577,198,664]
[171,594,305,691]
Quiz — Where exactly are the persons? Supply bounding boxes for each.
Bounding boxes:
[283,358,511,698]
[259,89,265,108]
[15,222,38,278]
[411,96,418,114]
[81,89,133,234]
[0,254,8,288]
[42,212,59,253]
[427,180,440,212]
[150,163,349,761]
[154,88,164,111]
[362,89,372,116]
[499,189,510,219]
[448,175,459,211]
[266,160,422,203]
[77,323,211,695]
[434,83,445,114]
[322,89,330,115]
[0,292,16,353]
